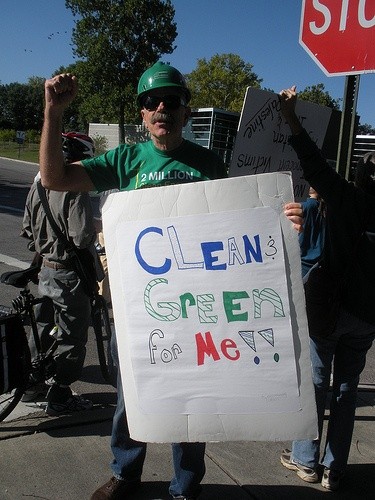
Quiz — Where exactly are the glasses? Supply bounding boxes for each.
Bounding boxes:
[144,96,188,110]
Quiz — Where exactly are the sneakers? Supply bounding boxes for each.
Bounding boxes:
[321,466,349,489]
[280,448,320,482]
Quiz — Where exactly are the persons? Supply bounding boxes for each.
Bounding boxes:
[19,128,98,412]
[279,80,375,489]
[38,63,304,500]
[295,181,330,280]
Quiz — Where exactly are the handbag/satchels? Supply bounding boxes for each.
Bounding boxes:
[68,246,101,298]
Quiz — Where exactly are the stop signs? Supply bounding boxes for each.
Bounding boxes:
[299,0,375,78]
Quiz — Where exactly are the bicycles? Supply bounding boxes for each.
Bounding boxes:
[0,243,115,425]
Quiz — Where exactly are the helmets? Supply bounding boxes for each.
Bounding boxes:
[136,64,192,105]
[63,132,95,164]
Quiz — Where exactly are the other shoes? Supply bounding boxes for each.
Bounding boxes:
[22,381,50,403]
[90,477,141,500]
[44,384,93,416]
